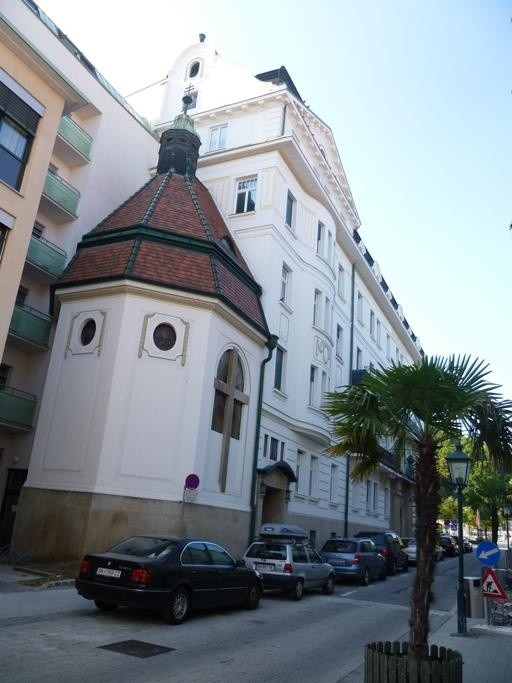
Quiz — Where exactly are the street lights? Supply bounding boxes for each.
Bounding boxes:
[446,441,473,635]
[503,499,511,569]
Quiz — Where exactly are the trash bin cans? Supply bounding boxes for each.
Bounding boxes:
[497,548,509,569]
[462,576,484,618]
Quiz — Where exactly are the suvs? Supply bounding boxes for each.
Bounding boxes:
[353,529,408,576]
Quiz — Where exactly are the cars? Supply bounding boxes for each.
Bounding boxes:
[75,534,265,625]
[401,535,473,567]
[242,538,336,601]
[320,536,388,586]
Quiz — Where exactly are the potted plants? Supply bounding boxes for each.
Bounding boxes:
[318,351,511,682]
